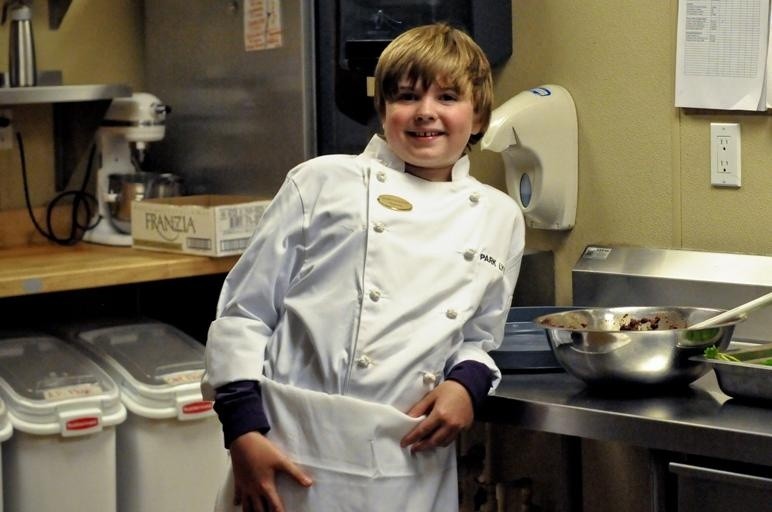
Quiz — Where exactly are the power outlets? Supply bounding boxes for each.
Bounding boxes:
[710,123,742,188]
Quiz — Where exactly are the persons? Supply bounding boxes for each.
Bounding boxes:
[199,23,525,510]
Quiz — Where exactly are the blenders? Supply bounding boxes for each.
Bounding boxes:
[81,92,169,246]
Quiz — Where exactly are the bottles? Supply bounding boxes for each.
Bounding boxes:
[9,5,37,87]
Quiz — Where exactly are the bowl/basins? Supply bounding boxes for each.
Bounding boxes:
[533,305,748,390]
[108,174,179,236]
